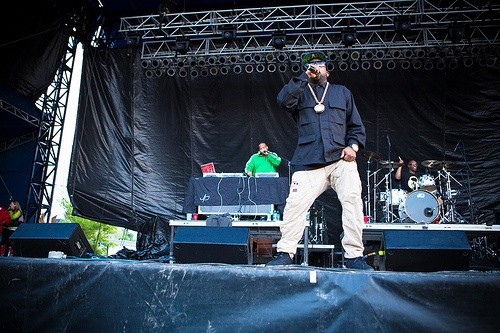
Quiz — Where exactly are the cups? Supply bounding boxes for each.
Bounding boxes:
[187,213,192,220]
[193,213,198,220]
[364,216,368,223]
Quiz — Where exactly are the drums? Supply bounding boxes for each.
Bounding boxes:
[398,190,440,224]
[389,189,406,208]
[417,174,437,193]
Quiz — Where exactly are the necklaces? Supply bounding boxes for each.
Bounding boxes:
[308,82,329,113]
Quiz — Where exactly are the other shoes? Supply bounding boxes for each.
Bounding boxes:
[265,252,292,267]
[343,256,375,273]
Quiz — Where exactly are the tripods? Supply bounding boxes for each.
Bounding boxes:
[439,168,469,225]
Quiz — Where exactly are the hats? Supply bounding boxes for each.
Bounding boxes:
[301,50,327,64]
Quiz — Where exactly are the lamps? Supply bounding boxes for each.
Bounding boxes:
[109,15,496,78]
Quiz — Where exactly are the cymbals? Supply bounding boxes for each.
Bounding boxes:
[421,159,444,169]
[361,150,380,161]
[379,160,402,169]
[431,160,457,170]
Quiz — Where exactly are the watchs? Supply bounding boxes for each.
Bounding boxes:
[349,143,359,152]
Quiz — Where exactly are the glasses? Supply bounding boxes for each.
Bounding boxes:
[304,60,326,69]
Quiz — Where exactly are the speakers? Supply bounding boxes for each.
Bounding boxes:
[377,230,473,272]
[9,223,94,258]
[174,227,252,266]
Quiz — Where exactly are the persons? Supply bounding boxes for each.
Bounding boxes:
[395,160,423,194]
[244,142,281,177]
[265,50,373,271]
[0,200,24,257]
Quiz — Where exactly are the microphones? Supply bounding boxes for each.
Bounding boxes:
[427,210,432,214]
[306,65,320,75]
[454,141,460,151]
[386,135,391,146]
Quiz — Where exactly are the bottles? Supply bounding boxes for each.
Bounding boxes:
[276,211,280,221]
[273,210,277,221]
